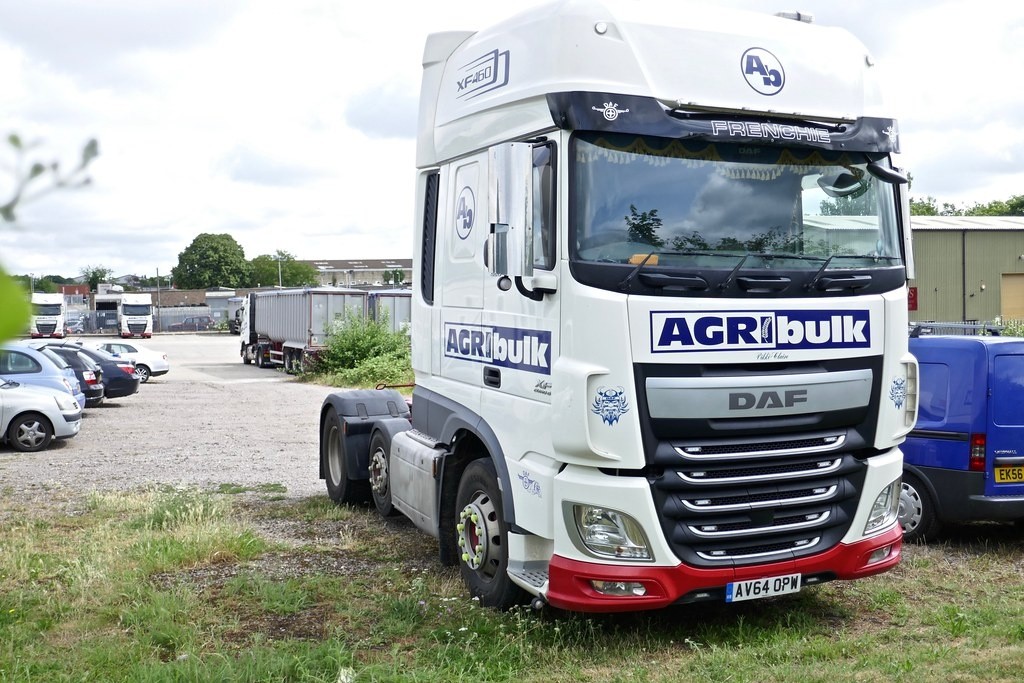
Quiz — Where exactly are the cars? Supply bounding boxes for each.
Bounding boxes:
[1,340,86,412]
[75,339,170,385]
[29,344,104,402]
[0,374,84,452]
[36,338,142,399]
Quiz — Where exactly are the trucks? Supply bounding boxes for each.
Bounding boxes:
[28,293,67,338]
[115,293,155,339]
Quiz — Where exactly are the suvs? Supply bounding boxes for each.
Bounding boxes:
[168,315,217,332]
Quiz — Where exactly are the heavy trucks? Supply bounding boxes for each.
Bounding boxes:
[317,0,921,615]
[234,283,369,376]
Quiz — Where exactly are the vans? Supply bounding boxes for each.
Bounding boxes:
[898,320,1024,545]
[65,320,85,335]
